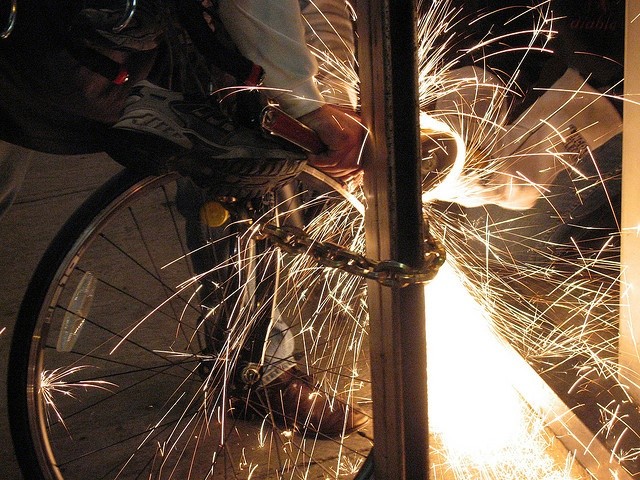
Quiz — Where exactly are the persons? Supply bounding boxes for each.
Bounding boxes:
[1,0,368,442]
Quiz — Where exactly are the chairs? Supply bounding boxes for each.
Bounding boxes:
[106,79,308,198]
[228,369,371,439]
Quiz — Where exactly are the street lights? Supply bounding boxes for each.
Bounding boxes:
[2,1,265,154]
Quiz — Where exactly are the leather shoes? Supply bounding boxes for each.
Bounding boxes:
[432,67,506,161]
[444,66,623,212]
